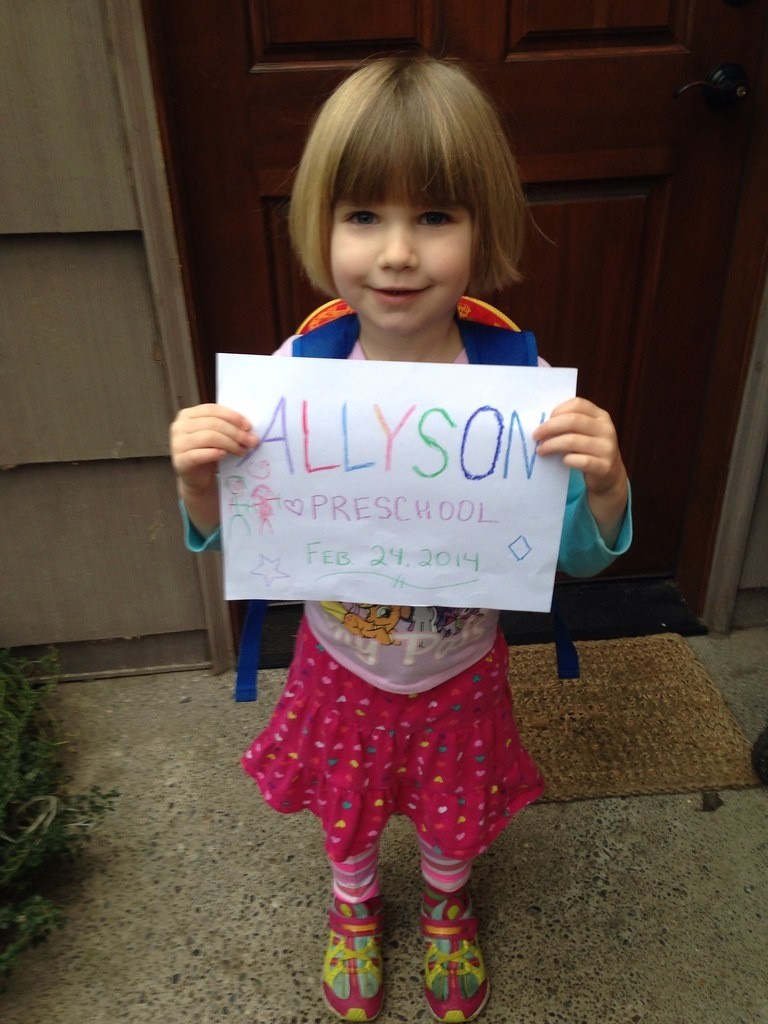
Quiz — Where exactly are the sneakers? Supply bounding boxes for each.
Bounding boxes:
[418,896,491,1023]
[320,896,385,1021]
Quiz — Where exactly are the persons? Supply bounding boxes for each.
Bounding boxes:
[167,56,634,1021]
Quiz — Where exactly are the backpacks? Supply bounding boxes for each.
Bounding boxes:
[233,292,581,704]
